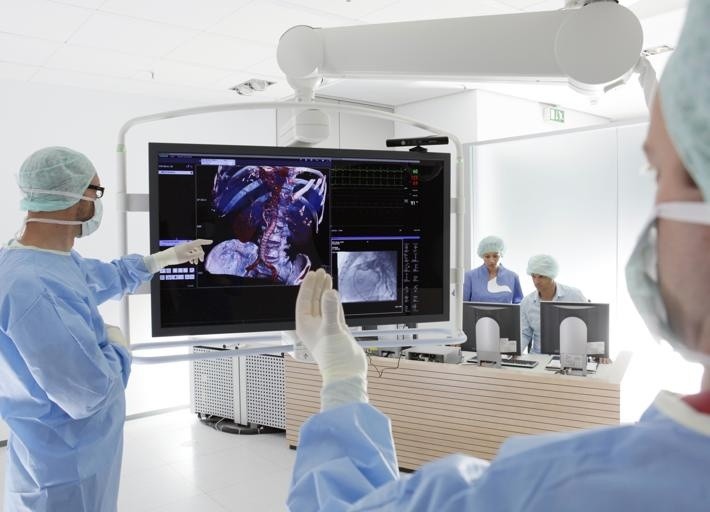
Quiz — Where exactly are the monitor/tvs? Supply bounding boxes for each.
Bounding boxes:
[539,301,610,375]
[148,142,452,338]
[462,301,522,369]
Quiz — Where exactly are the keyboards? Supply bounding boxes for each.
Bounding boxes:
[467,356,538,369]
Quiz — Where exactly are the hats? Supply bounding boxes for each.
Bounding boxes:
[655,0,710,196]
[477,234,505,257]
[18,146,97,215]
[527,255,560,279]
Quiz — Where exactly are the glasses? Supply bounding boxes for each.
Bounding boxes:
[87,182,104,200]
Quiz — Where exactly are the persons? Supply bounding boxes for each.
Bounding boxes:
[285,0,710,512]
[463,236,524,304]
[0,146,213,512]
[520,255,612,364]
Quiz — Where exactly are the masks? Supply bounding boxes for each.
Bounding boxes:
[624,220,707,361]
[70,196,102,239]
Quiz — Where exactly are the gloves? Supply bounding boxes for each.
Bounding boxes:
[144,239,213,275]
[103,322,129,352]
[296,268,369,413]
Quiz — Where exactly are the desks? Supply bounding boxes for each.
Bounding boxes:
[282,349,624,476]
